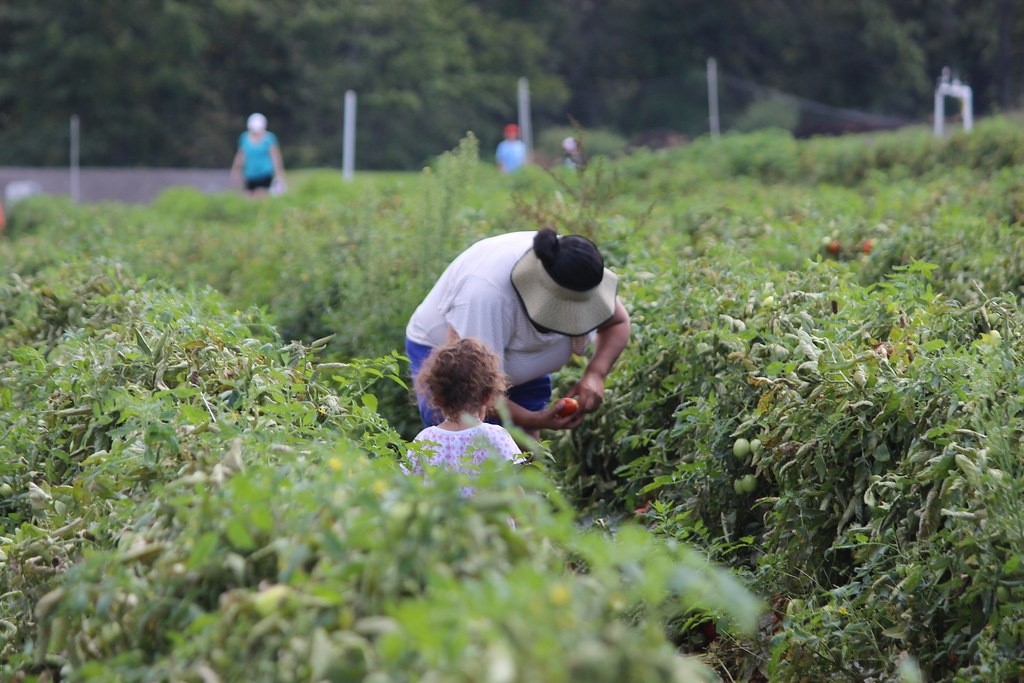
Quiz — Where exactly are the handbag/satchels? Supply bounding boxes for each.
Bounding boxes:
[569,331,591,356]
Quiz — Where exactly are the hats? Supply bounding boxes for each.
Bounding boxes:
[248,114,266,132]
[504,123,520,138]
[562,137,578,154]
[511,247,619,337]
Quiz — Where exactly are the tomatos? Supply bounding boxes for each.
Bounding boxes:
[551,239,875,497]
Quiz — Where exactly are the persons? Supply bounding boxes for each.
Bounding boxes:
[400,338,526,526]
[561,137,582,171]
[404,229,630,436]
[495,124,527,174]
[230,113,286,197]
